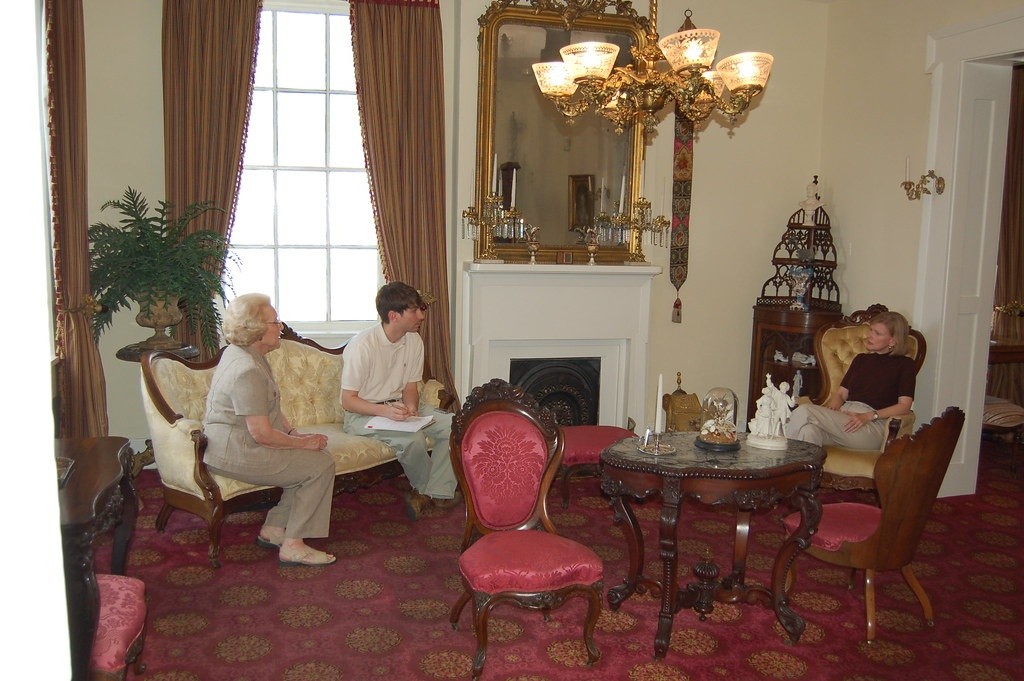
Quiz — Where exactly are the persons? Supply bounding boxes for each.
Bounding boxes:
[201,293,336,567]
[792,369,803,396]
[786,310,918,450]
[753,372,795,441]
[340,280,460,521]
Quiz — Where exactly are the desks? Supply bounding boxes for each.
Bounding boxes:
[54,437,140,681]
[115,344,200,478]
[988,336,1024,365]
[599,432,825,661]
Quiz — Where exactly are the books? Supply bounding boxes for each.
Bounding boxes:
[363,413,435,433]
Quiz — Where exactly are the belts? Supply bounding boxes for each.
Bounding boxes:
[376,400,399,404]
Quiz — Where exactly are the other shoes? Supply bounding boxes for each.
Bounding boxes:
[403,491,427,520]
[434,492,462,512]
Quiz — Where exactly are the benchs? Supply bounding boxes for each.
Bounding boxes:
[141,322,456,568]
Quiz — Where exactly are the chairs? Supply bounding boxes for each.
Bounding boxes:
[982,395,1024,473]
[780,405,966,649]
[788,303,928,491]
[449,378,605,681]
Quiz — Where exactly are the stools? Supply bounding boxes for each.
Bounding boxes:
[560,426,639,510]
[90,574,146,681]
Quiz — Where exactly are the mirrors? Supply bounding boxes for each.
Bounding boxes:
[473,0,645,265]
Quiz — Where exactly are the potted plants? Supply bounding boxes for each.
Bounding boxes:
[86,184,243,361]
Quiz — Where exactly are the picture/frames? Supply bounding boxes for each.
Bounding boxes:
[567,175,595,232]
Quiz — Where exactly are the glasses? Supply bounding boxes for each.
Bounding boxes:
[264,320,282,327]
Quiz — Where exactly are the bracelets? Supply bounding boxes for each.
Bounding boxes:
[287,427,297,435]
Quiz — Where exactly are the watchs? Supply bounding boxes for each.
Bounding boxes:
[872,410,879,422]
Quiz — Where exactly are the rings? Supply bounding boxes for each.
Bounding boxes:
[854,424,858,427]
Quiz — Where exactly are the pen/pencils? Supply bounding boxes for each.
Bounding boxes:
[385,401,405,410]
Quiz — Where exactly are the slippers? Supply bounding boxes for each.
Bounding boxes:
[279,546,336,565]
[255,536,280,549]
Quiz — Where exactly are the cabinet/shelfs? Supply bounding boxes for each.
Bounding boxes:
[745,171,844,433]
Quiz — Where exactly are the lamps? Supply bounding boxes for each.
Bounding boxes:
[531,0,774,137]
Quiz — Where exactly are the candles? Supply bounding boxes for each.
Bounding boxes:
[641,160,645,197]
[601,177,606,212]
[492,153,497,192]
[619,175,625,213]
[498,170,503,196]
[660,176,664,216]
[510,168,516,211]
[906,156,910,182]
[655,373,663,433]
[470,169,474,207]
